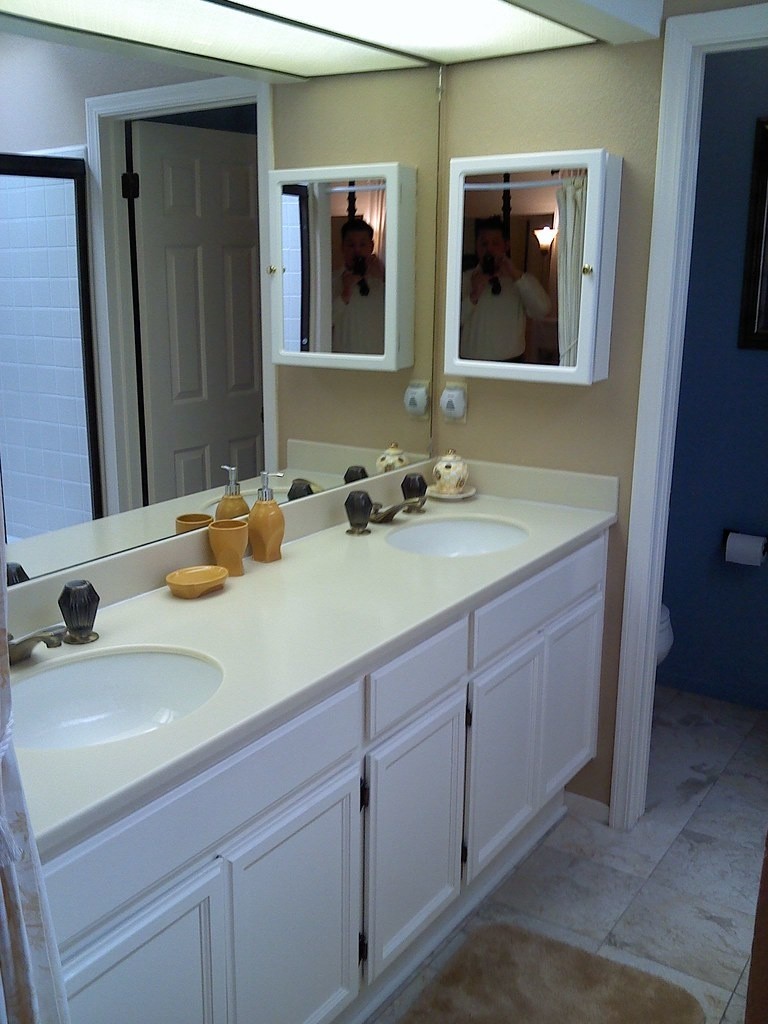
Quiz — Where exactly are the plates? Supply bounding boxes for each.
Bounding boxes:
[426,485,476,501]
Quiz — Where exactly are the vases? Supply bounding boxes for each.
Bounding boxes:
[56,578,101,647]
[401,472,428,514]
[345,491,373,536]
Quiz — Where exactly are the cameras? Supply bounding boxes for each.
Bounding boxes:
[481,254,496,273]
[350,254,369,276]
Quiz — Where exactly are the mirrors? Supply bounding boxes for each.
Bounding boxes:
[266,161,420,373]
[0,0,444,599]
[439,147,625,388]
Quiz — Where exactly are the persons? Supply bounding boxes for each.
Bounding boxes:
[332,220,386,356]
[461,210,552,364]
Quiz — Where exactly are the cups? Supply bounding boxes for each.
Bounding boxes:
[176,513,214,535]
[208,520,248,577]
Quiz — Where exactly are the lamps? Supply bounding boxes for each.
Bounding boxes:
[533,225,559,294]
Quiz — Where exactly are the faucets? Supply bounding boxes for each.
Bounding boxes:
[369,496,426,524]
[4,625,68,663]
[293,476,323,494]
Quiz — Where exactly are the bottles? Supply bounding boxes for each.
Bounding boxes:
[375,443,410,474]
[432,449,470,494]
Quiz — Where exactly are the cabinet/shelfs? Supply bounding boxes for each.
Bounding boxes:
[471,525,612,897]
[365,609,465,994]
[39,671,363,1024]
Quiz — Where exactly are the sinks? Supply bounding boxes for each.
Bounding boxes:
[387,514,529,559]
[9,644,225,754]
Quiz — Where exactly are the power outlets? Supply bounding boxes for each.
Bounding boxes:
[440,379,471,424]
[407,379,431,421]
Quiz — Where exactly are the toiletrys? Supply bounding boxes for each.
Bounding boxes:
[215,464,250,523]
[249,471,288,563]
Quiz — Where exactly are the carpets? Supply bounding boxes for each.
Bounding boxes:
[396,918,711,1024]
